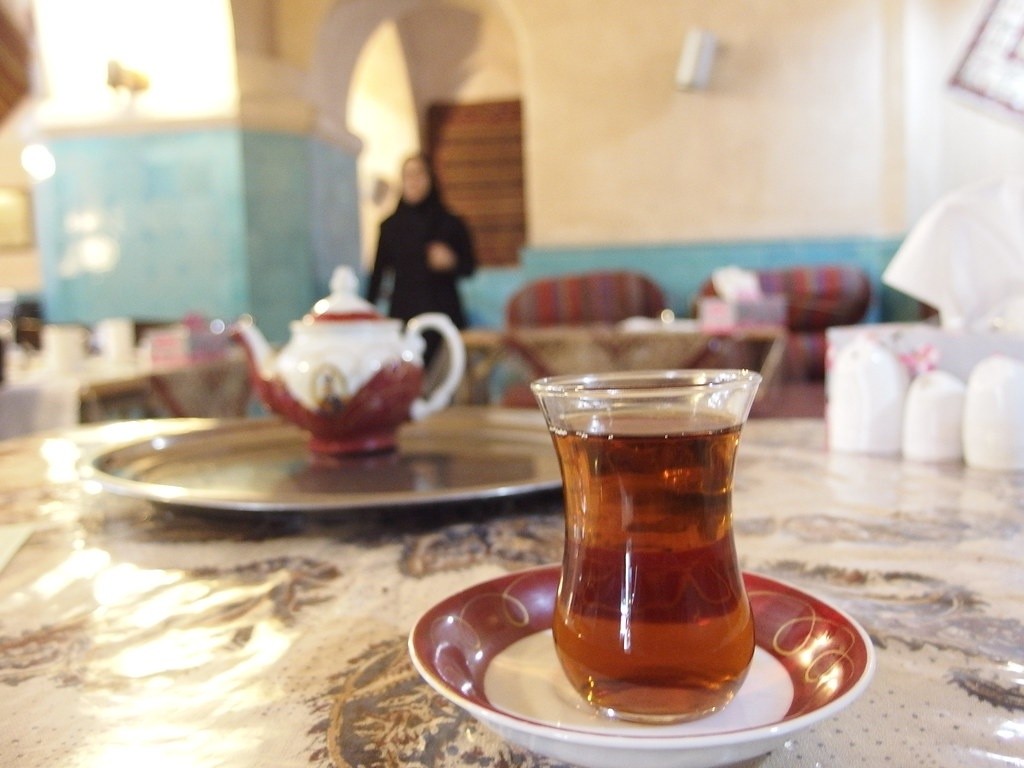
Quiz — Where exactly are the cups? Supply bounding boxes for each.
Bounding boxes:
[825,342,1024,473]
[39,324,87,372]
[530,369,763,726]
[93,317,136,362]
[0,320,13,388]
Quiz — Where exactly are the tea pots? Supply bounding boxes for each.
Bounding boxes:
[227,265,468,457]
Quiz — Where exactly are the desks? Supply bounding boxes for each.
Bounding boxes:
[0,413,1024,768]
[426,320,788,417]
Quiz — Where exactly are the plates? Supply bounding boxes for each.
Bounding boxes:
[408,563,877,768]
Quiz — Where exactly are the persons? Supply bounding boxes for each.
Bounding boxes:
[362,153,479,370]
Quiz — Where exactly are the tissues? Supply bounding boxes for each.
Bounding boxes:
[696,266,780,333]
[821,184,1022,457]
